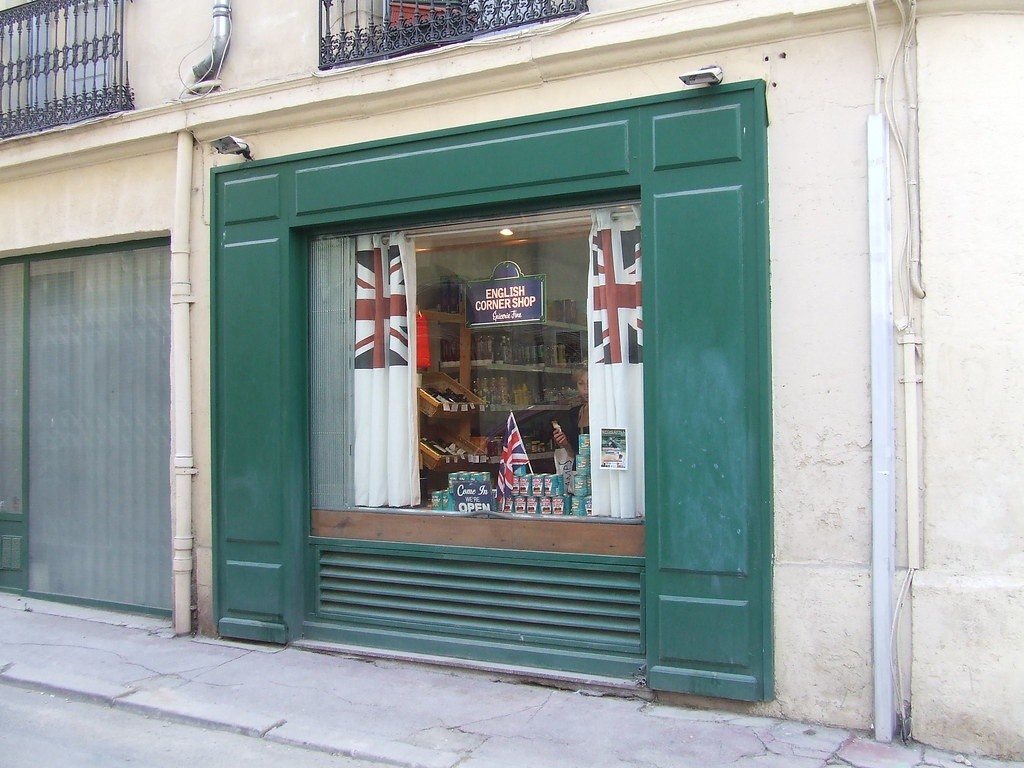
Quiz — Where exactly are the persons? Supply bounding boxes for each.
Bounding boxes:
[552,359,589,496]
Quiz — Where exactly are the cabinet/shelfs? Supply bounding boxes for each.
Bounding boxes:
[416,284,587,506]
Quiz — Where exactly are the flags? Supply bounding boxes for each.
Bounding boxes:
[494,414,530,499]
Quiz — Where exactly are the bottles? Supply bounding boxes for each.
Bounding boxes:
[420,274,585,405]
[420,434,470,455]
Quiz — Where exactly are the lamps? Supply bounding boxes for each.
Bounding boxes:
[210,135,255,161]
[678,64,723,88]
